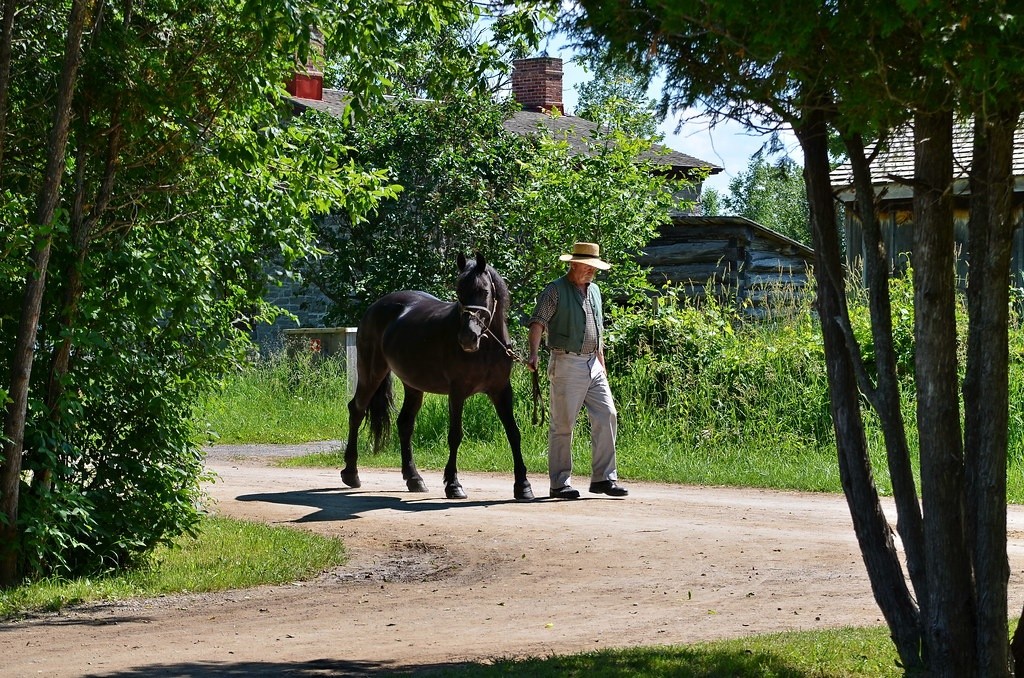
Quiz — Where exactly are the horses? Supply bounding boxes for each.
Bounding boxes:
[341,251,535,502]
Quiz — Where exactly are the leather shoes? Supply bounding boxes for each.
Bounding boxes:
[549,486,580,499]
[589,480,628,496]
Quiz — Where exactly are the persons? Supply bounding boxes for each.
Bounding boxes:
[529,243,628,499]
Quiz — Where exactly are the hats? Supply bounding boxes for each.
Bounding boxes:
[558,242,611,270]
